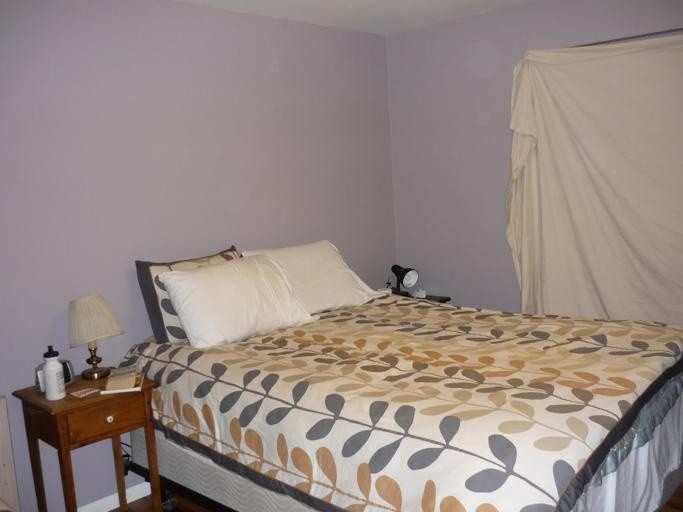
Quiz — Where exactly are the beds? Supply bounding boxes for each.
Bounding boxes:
[119,295,683,510]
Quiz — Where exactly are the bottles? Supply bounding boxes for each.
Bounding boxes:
[42,345,66,401]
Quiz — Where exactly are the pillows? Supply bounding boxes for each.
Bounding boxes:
[134,235,380,350]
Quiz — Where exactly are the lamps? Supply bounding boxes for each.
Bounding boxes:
[66,291,123,383]
[388,263,419,298]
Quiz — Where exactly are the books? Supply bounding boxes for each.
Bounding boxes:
[64,382,99,399]
[98,363,144,395]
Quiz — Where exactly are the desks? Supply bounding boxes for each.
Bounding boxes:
[11,372,165,511]
